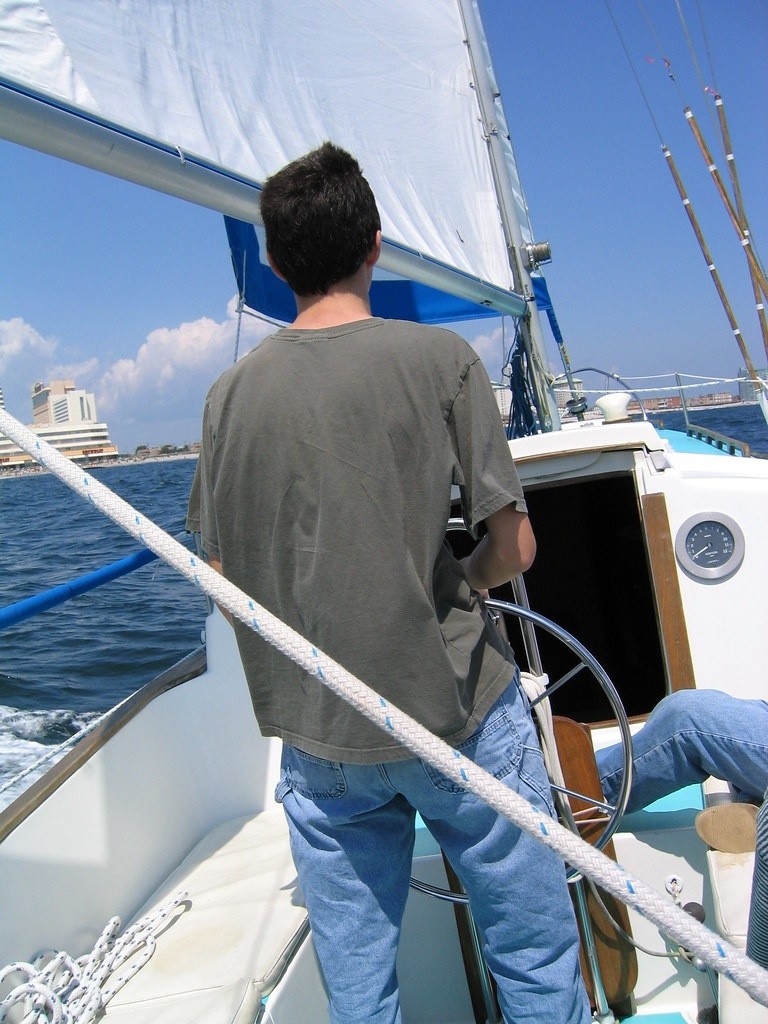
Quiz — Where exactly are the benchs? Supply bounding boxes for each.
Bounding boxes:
[704,775,761,947]
[71,812,334,1024]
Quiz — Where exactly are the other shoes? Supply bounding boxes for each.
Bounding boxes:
[695,801,761,853]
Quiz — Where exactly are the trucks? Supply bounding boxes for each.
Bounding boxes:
[658,402,668,409]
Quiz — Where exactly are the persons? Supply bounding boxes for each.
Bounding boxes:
[590,688,768,820]
[186,140,593,1024]
